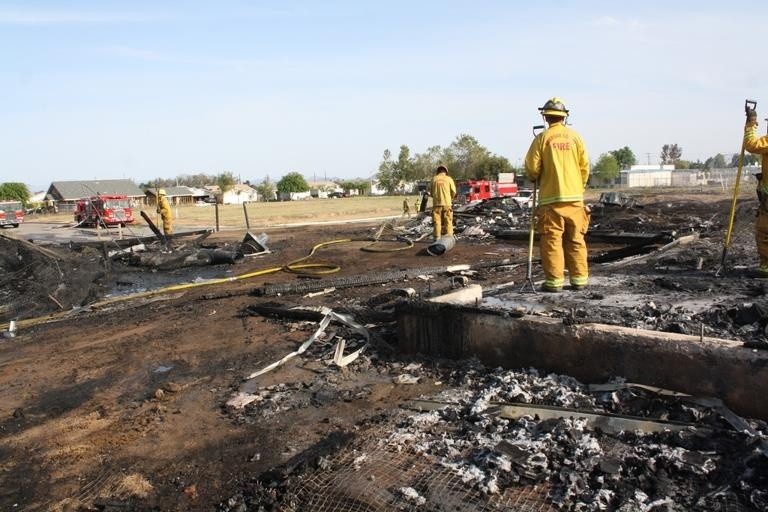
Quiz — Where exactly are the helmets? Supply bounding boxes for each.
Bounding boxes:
[542,97,569,117]
[159,189,167,196]
[437,166,448,175]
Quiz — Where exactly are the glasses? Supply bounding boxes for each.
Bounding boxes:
[542,100,567,111]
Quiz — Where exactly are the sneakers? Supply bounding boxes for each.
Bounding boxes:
[542,282,586,292]
[752,266,768,274]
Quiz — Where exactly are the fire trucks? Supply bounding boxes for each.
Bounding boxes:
[0,200,24,228]
[449,181,518,205]
[74,193,134,228]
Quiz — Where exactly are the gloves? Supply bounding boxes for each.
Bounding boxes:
[746,106,758,127]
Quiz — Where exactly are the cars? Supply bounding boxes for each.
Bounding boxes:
[328,192,344,198]
[205,198,217,203]
[512,190,538,210]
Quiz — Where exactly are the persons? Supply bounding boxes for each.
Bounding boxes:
[744,106,768,276]
[430,164,457,243]
[402,197,411,219]
[156,188,173,242]
[526,96,591,292]
[415,199,420,214]
